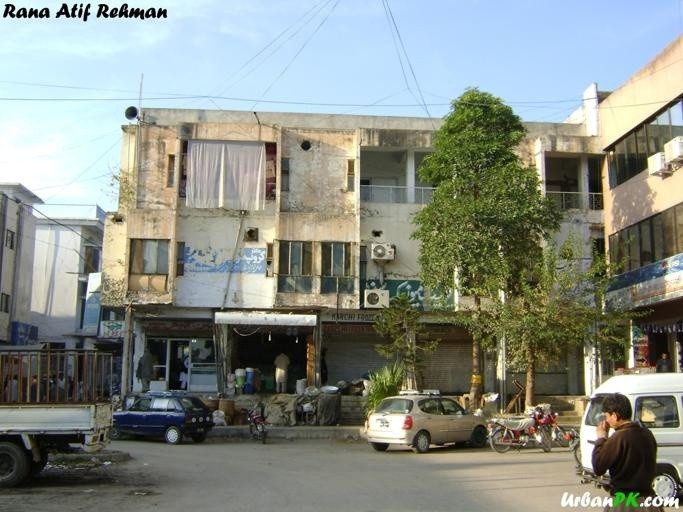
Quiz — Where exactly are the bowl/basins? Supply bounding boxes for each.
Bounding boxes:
[320,385,339,394]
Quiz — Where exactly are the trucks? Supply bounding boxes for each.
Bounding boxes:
[0,352,113,487]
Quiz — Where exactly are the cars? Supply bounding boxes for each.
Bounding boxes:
[367,395,487,453]
[109,391,212,444]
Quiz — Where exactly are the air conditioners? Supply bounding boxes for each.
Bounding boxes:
[647,136,683,176]
[370,242,396,260]
[364,289,390,308]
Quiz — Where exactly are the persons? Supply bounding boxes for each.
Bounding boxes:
[592,394,656,512]
[656,352,673,373]
[274,351,290,393]
[136,347,154,392]
[4,373,76,403]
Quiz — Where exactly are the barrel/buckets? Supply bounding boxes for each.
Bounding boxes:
[217,399,234,423]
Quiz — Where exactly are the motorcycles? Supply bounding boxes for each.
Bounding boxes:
[488,404,573,453]
[240,401,272,444]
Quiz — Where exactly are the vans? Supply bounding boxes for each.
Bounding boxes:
[580,372,683,498]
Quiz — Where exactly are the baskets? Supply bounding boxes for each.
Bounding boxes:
[202,399,247,425]
